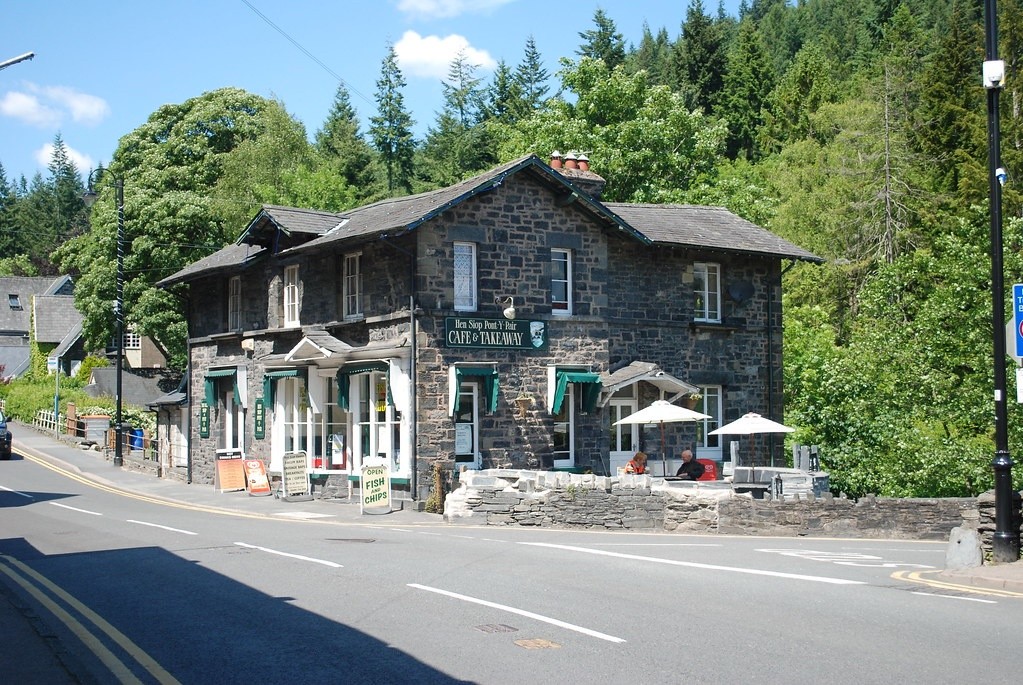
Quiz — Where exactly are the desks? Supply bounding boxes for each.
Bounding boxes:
[651,476,682,480]
[733,481,771,499]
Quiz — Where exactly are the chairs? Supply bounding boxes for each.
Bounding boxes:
[695,458,717,483]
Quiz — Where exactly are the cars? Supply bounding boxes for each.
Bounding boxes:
[0,411,13,459]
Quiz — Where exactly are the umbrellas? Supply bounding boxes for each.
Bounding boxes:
[707,412,795,483]
[612,400,714,476]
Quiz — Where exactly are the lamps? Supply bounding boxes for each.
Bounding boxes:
[241,338,254,351]
[494,296,516,319]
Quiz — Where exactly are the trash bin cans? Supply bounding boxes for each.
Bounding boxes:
[131,428,143,450]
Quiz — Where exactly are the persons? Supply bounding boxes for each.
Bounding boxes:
[676,450,705,481]
[624,452,647,475]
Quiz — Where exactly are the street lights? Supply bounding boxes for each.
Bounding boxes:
[82,168,124,465]
